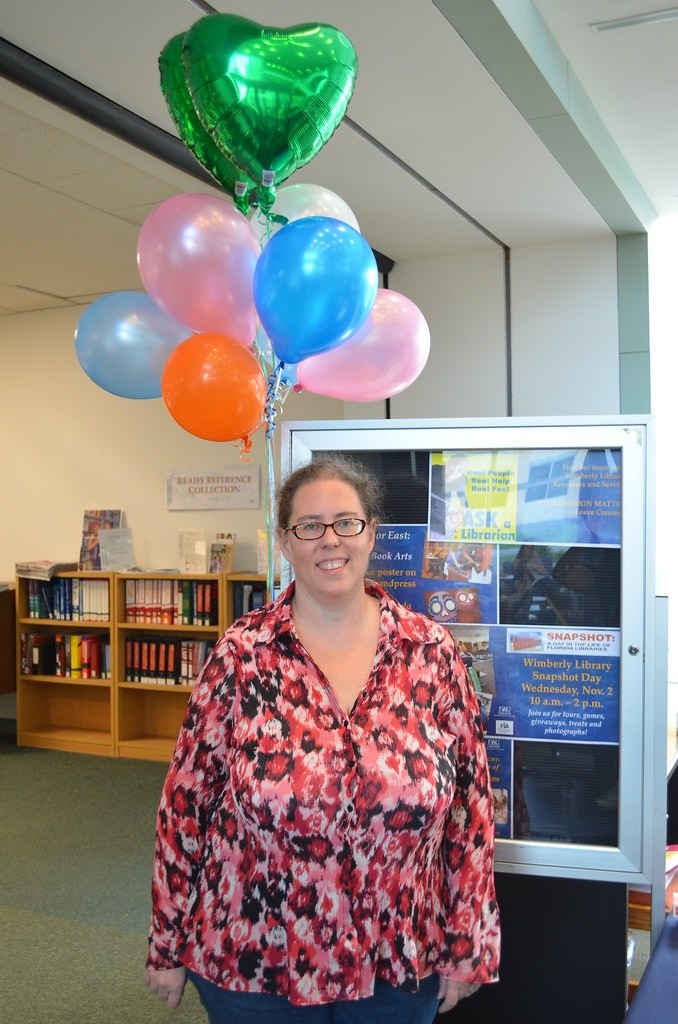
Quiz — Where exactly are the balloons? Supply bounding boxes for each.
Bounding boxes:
[251,183,361,250]
[253,215,378,364]
[159,13,359,213]
[136,193,262,347]
[257,288,430,401]
[75,291,200,399]
[163,332,270,443]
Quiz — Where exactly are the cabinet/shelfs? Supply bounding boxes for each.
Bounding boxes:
[15,570,282,763]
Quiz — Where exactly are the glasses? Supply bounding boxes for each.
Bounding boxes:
[286,518,370,541]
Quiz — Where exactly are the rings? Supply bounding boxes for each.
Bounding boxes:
[468,990,474,993]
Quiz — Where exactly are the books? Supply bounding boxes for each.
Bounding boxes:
[125,580,219,626]
[233,582,281,623]
[29,578,109,622]
[77,509,121,571]
[126,635,218,686]
[209,532,236,574]
[19,632,110,680]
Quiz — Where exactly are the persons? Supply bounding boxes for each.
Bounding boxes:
[144,458,500,1024]
[501,544,620,627]
[444,542,494,581]
